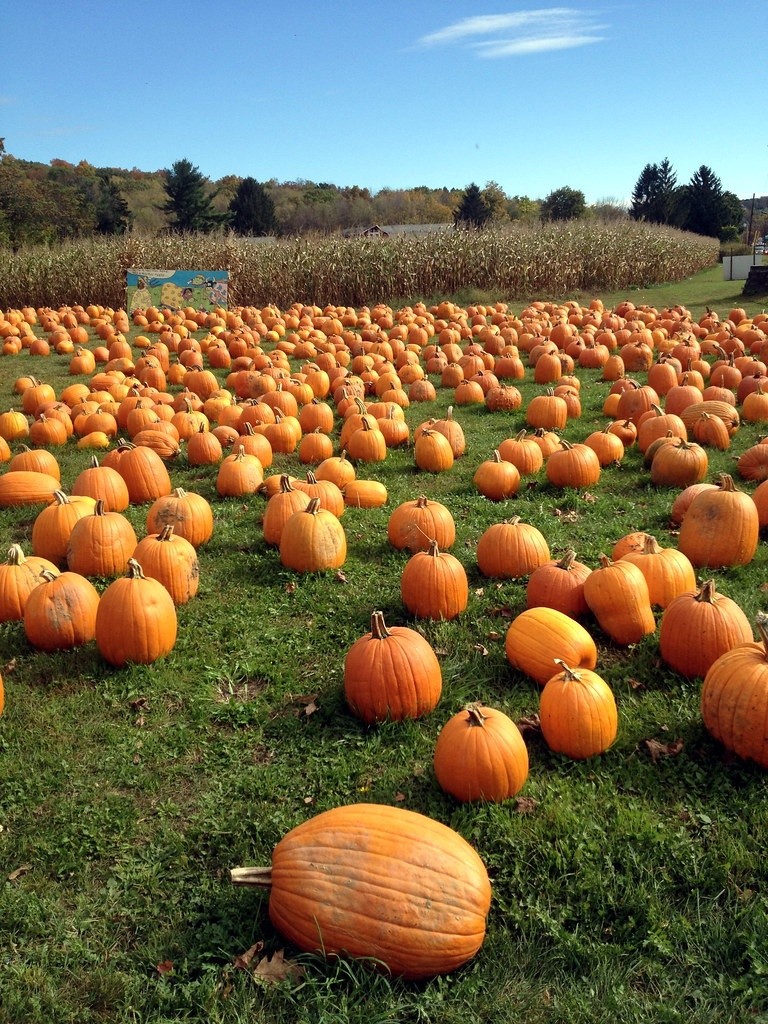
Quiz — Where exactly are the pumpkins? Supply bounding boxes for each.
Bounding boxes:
[229,803,492,975]
[0,299,768,799]
